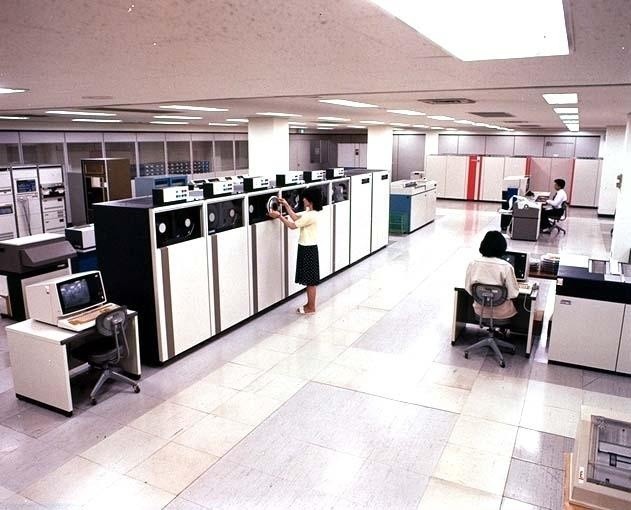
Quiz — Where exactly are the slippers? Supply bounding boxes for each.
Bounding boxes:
[297,310,316,315]
[297,306,306,310]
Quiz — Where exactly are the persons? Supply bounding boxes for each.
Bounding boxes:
[540,179,567,234]
[465,231,519,337]
[269,188,322,314]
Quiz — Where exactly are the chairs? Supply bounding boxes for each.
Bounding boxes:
[464,282,516,368]
[547,201,567,235]
[70,305,140,405]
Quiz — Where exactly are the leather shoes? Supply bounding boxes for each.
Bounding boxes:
[541,229,551,233]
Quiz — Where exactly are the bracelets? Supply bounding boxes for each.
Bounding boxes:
[278,214,281,218]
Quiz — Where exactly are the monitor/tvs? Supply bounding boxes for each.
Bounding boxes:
[503,176,530,196]
[25,270,107,327]
[15,178,37,196]
[500,251,531,281]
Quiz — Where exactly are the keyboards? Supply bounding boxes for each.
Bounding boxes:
[58,302,121,332]
[517,282,528,289]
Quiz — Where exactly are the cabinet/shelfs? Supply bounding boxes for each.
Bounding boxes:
[548,294,631,379]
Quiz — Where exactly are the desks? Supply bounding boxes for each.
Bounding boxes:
[5,309,141,417]
[511,192,551,241]
[452,281,539,358]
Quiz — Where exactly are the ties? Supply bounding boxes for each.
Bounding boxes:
[552,193,557,208]
